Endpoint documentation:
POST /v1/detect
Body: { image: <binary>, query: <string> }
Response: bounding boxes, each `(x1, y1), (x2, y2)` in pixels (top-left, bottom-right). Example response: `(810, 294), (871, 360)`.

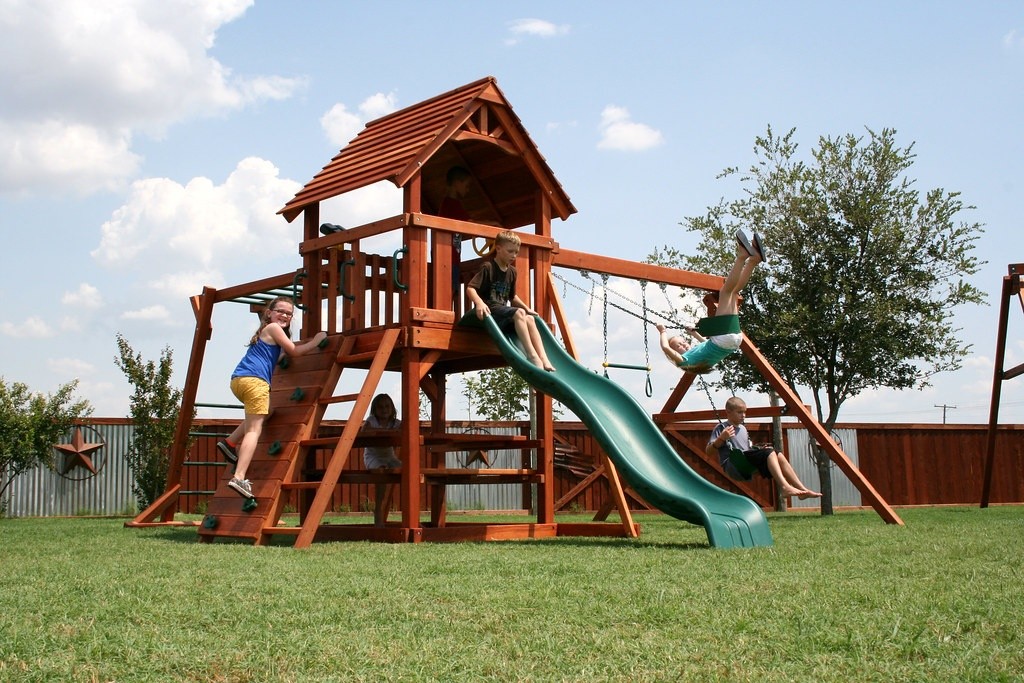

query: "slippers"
(752, 233), (766, 262)
(736, 229), (755, 256)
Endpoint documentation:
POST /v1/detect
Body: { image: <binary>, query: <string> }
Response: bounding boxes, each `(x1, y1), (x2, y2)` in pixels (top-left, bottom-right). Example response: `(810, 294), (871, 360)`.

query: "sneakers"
(227, 478), (255, 499)
(217, 439), (238, 464)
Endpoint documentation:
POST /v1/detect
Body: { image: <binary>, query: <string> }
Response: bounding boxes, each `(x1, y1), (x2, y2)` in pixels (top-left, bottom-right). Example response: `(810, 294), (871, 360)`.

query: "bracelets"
(718, 437), (725, 443)
(661, 329), (667, 333)
(712, 443), (719, 450)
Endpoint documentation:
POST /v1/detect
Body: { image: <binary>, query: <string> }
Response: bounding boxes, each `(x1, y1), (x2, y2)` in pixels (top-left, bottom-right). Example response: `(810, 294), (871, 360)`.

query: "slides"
(459, 303), (774, 549)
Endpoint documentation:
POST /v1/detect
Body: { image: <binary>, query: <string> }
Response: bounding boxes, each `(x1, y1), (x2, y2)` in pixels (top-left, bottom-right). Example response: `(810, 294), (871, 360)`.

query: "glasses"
(271, 310), (293, 318)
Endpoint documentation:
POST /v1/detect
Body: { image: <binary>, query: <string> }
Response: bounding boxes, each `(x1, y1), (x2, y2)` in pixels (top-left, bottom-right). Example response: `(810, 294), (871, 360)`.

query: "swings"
(550, 269), (742, 337)
(658, 284), (758, 482)
(602, 273), (652, 371)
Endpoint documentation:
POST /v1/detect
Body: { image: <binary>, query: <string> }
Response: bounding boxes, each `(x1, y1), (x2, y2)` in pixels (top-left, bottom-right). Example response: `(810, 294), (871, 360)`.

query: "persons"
(654, 228), (767, 373)
(362, 393), (408, 525)
(465, 230), (557, 372)
(216, 295), (327, 499)
(703, 397), (823, 500)
